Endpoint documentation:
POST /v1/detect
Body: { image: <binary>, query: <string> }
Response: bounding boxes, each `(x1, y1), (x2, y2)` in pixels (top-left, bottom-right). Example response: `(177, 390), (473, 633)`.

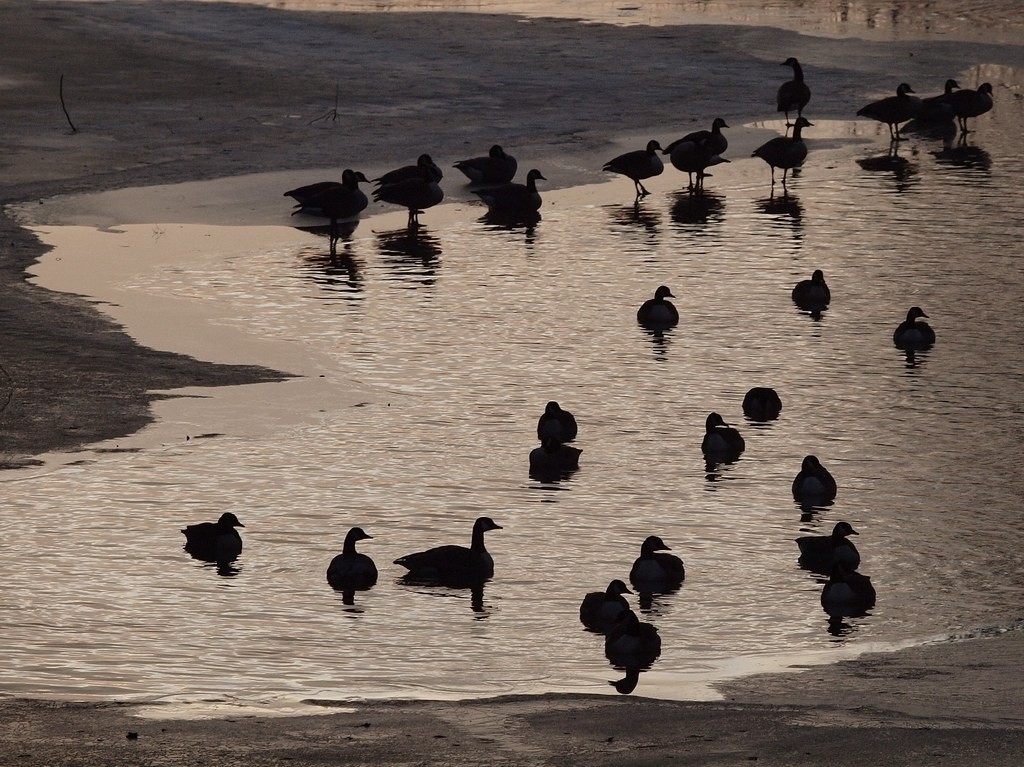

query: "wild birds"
(601, 140), (665, 200)
(371, 154), (445, 214)
(629, 533), (687, 597)
(742, 387), (783, 420)
(792, 269), (831, 311)
(637, 285), (680, 328)
(792, 521), (862, 574)
(792, 455), (838, 506)
(283, 168), (372, 220)
(577, 580), (639, 632)
(663, 117), (731, 191)
(603, 624), (663, 697)
(452, 144), (547, 217)
(893, 306), (936, 350)
(701, 413), (745, 463)
(819, 575), (878, 638)
(856, 78), (995, 142)
(327, 528), (379, 590)
(178, 512), (247, 563)
(392, 515), (506, 581)
(776, 57), (816, 128)
(537, 401), (578, 443)
(750, 117), (815, 193)
